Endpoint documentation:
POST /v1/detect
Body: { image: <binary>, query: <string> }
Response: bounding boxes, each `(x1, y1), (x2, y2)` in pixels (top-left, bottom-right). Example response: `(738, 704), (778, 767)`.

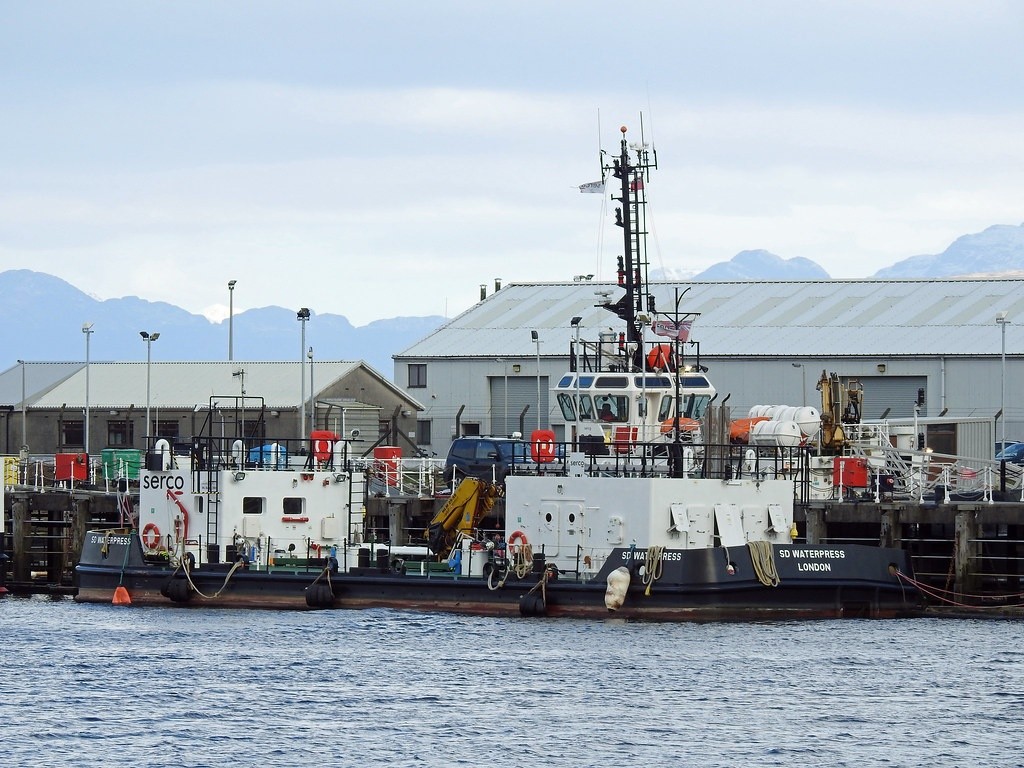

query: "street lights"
(570, 316), (583, 453)
(296, 306), (310, 451)
(138, 331), (160, 453)
(82, 320), (95, 454)
(991, 309), (1012, 462)
(233, 369), (246, 438)
(226, 279), (238, 360)
(531, 330), (544, 431)
(16, 359), (26, 447)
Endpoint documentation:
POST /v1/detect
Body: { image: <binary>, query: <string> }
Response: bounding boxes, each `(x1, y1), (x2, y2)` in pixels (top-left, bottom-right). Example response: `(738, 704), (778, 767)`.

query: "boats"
(74, 104), (1022, 622)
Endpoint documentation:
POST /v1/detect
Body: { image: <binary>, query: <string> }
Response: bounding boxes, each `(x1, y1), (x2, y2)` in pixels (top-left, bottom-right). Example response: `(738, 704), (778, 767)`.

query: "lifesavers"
(142, 522), (161, 550)
(303, 585), (333, 609)
(389, 558), (406, 578)
(507, 530), (528, 556)
